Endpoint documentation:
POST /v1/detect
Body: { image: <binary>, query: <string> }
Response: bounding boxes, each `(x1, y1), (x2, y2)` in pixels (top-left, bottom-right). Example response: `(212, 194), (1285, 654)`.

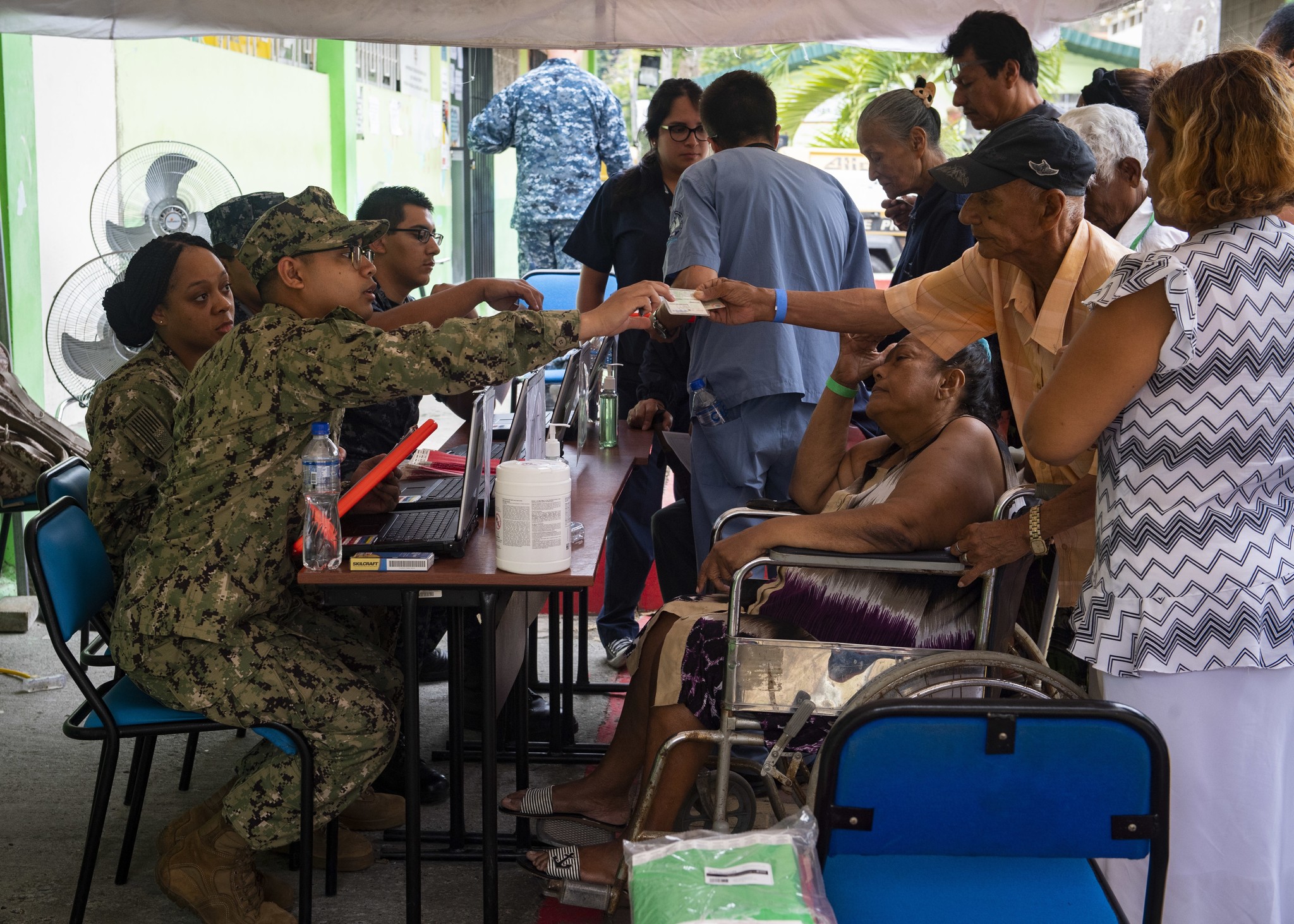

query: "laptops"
(342, 335), (614, 556)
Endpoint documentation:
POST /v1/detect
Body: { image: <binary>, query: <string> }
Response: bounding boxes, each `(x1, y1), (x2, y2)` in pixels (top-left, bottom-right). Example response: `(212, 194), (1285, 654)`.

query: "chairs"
(24, 456), (338, 924)
(511, 269), (617, 413)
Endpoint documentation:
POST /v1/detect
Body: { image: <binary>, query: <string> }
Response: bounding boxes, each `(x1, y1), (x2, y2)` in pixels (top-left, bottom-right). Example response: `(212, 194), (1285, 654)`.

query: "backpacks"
(0, 340), (92, 509)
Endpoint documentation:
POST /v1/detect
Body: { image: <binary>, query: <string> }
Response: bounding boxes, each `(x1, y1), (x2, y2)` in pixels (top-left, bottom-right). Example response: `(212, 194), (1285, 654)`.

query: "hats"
(204, 191), (292, 249)
(235, 185), (389, 287)
(928, 114), (1098, 197)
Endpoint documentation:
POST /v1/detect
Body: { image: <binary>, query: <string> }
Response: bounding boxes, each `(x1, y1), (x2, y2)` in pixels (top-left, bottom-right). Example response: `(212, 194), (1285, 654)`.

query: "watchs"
(1029, 504), (1050, 557)
(653, 308), (677, 339)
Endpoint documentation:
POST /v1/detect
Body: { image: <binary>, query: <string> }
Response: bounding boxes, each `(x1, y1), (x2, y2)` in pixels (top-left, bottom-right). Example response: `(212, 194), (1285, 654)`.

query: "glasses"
(944, 55), (1010, 83)
(706, 134), (718, 143)
(660, 124), (709, 141)
(388, 227), (444, 246)
(290, 243), (375, 271)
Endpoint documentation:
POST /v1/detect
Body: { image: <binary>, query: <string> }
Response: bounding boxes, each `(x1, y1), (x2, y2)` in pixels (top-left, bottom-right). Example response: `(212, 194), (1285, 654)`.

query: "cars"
(508, 267), (625, 419)
(857, 231), (903, 295)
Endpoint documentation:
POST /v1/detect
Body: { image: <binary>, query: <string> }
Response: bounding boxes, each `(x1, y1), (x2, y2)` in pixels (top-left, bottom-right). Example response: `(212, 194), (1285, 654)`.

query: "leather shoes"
(372, 731), (450, 804)
(419, 647), (448, 681)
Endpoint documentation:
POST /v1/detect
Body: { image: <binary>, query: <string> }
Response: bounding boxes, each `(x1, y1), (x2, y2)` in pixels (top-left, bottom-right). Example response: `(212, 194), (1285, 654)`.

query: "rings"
(956, 540), (965, 554)
(962, 553), (969, 563)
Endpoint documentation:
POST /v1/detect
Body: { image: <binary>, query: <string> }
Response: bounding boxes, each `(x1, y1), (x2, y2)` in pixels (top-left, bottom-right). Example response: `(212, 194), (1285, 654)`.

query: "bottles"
(603, 347), (612, 368)
(689, 378), (727, 427)
(590, 336), (604, 370)
(301, 423), (342, 570)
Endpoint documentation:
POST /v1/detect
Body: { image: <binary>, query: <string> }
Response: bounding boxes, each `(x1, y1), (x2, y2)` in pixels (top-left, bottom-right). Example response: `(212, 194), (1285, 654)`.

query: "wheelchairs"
(535, 479), (1108, 915)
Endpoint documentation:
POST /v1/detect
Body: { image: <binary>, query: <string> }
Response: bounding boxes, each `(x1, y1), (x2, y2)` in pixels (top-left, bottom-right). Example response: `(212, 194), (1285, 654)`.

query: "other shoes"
(269, 821), (375, 871)
(338, 786), (406, 830)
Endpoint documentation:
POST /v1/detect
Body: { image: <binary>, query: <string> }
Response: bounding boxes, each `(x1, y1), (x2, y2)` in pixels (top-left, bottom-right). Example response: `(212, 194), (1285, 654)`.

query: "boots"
(153, 809), (299, 924)
(157, 774), (295, 912)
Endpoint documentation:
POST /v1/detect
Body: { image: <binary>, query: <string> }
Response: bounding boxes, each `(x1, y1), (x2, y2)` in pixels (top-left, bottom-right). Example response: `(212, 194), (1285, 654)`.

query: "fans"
(47, 141), (243, 408)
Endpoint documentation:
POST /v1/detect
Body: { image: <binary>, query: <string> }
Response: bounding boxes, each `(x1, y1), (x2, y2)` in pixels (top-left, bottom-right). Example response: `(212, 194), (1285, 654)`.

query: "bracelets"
(773, 288), (788, 324)
(825, 376), (860, 399)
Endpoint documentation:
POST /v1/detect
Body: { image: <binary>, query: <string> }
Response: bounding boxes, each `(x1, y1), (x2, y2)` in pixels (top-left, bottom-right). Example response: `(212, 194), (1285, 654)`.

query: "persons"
(463, 1), (1294, 923)
(86, 181), (676, 924)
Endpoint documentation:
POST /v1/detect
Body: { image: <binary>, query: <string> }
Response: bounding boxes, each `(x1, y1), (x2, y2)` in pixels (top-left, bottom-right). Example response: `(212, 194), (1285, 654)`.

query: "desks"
(297, 420), (654, 924)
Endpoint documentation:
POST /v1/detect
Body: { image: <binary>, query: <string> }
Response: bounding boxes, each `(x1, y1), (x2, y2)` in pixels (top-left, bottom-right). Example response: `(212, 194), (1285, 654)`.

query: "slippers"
(498, 785), (628, 833)
(517, 845), (629, 894)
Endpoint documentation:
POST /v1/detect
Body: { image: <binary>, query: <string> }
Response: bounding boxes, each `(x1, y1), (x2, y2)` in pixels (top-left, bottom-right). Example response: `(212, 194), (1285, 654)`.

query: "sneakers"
(604, 636), (634, 668)
